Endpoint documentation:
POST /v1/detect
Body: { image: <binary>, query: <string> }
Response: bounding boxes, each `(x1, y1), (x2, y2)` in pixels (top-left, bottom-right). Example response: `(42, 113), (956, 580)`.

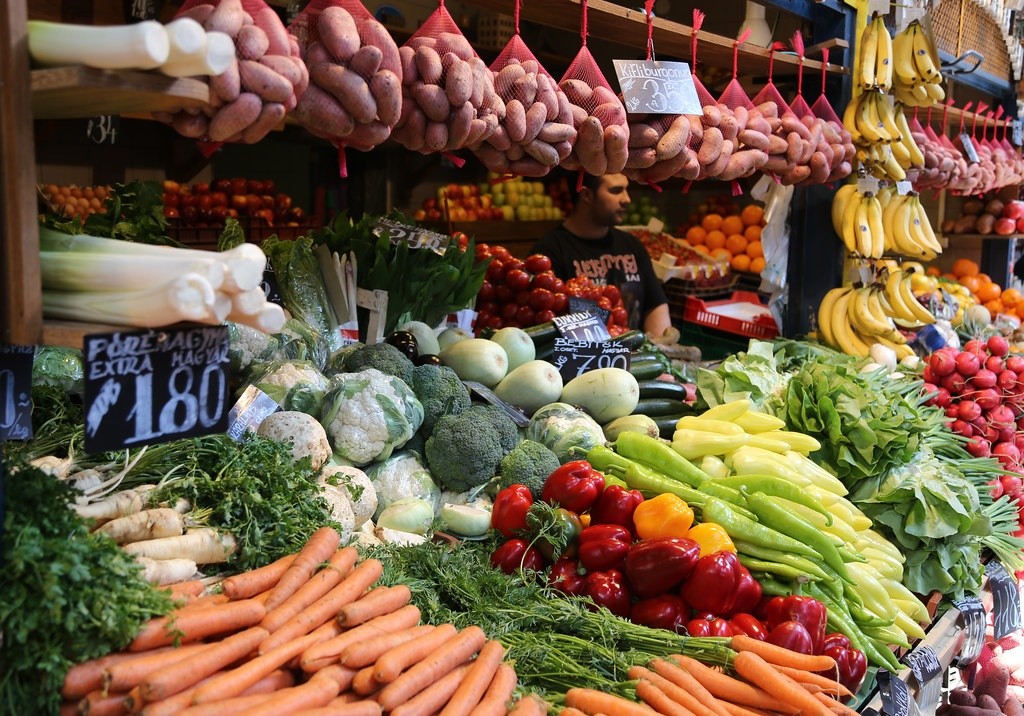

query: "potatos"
(942, 197), (1004, 235)
(936, 628), (1024, 716)
(909, 132), (1024, 196)
(168, 0), (855, 186)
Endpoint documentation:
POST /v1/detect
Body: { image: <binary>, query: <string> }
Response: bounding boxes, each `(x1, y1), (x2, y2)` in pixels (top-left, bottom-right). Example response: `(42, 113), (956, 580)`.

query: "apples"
(163, 179), (305, 225)
(413, 174), (573, 222)
(620, 195), (740, 238)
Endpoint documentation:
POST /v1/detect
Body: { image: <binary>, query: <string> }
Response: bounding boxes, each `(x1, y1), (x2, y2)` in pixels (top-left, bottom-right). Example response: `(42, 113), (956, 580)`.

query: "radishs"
(10, 456), (236, 589)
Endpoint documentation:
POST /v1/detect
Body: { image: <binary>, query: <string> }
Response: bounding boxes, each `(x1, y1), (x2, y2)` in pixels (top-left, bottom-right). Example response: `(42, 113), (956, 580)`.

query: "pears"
(41, 182), (112, 220)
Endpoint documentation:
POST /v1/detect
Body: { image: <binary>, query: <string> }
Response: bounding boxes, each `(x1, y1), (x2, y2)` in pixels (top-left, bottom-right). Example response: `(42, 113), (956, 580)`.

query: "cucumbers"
(515, 321), (714, 440)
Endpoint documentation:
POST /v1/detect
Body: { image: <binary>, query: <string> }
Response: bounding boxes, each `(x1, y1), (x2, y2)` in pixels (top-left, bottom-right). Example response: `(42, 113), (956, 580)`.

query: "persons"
(526, 170), (671, 337)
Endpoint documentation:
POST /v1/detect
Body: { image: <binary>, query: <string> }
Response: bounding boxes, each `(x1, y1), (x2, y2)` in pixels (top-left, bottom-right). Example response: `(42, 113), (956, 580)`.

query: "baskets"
(682, 291), (781, 340)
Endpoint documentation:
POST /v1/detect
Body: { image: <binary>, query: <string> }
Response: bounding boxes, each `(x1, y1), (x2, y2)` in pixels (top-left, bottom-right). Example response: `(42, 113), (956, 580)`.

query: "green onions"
(26, 19), (234, 77)
(38, 226), (286, 334)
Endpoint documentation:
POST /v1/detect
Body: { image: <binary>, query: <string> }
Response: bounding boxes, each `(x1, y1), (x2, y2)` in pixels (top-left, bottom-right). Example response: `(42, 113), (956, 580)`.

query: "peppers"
(493, 399), (934, 701)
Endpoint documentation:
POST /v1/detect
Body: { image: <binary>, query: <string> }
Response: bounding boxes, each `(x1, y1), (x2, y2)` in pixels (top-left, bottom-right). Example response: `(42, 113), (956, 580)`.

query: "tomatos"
(449, 231), (629, 339)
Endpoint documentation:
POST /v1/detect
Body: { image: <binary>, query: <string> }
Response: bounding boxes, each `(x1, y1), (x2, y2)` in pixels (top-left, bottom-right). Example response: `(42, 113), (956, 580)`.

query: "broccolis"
(227, 323), (561, 551)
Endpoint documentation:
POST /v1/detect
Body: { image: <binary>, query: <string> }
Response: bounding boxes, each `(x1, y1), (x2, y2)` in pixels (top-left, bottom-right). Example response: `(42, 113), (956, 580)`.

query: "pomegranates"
(993, 196), (1024, 236)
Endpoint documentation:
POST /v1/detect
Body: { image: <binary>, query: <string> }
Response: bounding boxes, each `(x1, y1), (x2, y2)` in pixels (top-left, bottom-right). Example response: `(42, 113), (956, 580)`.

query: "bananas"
(814, 9), (944, 361)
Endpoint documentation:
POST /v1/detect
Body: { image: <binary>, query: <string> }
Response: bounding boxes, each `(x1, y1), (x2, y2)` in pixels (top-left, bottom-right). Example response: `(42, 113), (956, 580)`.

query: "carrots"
(59, 527), (859, 716)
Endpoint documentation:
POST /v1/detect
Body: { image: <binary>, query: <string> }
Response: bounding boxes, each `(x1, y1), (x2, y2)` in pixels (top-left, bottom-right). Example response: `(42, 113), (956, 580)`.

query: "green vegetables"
(692, 338), (1024, 601)
(47, 177), (189, 249)
(217, 208), (493, 346)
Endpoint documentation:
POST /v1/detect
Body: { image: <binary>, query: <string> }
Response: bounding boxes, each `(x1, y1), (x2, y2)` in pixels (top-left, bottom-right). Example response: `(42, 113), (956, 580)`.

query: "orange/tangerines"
(933, 258), (1024, 341)
(676, 205), (767, 277)
